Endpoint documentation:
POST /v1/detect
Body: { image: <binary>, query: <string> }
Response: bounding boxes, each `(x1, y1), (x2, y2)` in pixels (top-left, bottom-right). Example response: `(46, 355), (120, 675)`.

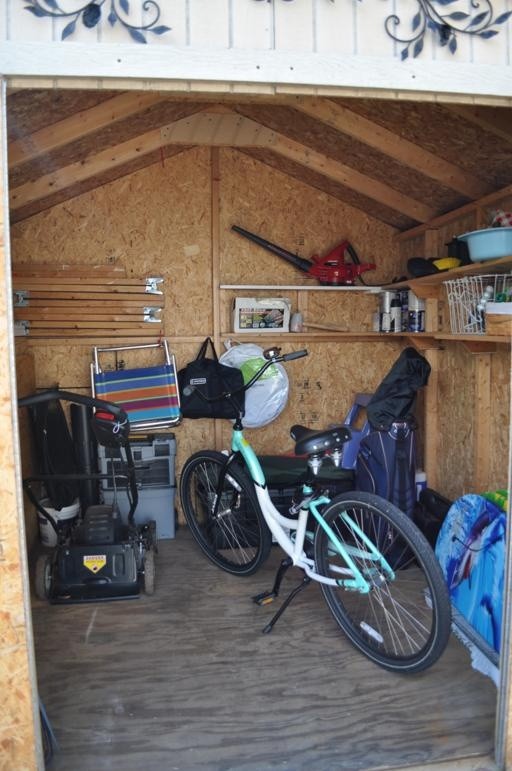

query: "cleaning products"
(17, 390), (155, 605)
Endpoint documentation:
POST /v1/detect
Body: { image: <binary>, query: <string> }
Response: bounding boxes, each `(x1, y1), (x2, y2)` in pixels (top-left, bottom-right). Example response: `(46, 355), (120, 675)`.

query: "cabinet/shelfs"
(390, 299), (402, 332)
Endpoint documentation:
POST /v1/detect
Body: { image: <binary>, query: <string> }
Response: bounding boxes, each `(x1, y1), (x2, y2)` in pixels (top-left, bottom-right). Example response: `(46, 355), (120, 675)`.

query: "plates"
(218, 255), (512, 349)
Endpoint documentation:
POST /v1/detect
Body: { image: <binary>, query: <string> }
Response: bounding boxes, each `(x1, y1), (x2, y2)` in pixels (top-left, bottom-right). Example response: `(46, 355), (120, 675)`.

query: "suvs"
(35, 499), (80, 547)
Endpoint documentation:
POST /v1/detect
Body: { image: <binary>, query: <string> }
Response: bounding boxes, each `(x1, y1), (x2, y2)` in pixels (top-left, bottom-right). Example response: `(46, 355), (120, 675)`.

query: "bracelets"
(456, 227), (512, 264)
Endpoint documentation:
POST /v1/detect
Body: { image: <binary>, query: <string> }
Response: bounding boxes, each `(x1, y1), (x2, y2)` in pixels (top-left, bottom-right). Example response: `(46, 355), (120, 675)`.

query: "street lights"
(177, 336), (247, 421)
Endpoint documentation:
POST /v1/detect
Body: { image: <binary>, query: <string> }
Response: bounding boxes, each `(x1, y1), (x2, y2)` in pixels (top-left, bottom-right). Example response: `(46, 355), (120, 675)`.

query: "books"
(204, 484), (340, 551)
(96, 432), (178, 542)
